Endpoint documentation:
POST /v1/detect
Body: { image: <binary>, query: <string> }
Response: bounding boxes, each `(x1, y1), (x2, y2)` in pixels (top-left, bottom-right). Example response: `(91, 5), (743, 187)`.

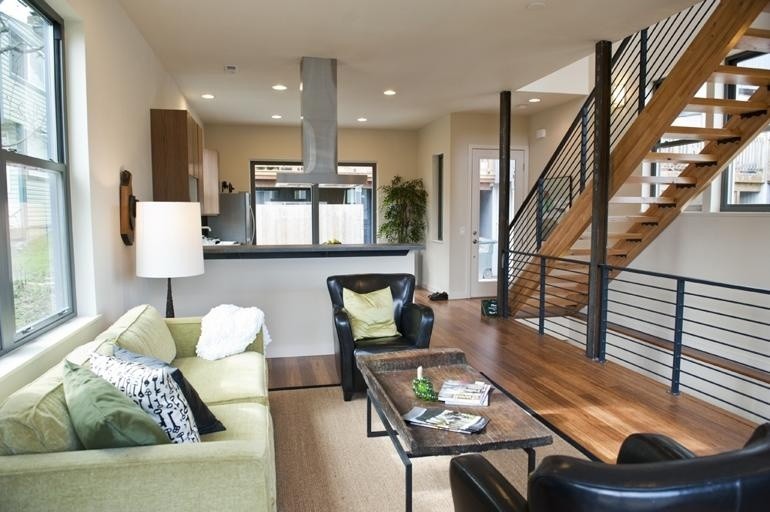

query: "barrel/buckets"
(481, 299), (497, 316)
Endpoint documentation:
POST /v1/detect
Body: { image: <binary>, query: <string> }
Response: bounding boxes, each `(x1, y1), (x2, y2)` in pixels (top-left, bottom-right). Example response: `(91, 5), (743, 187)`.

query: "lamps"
(131, 199), (209, 316)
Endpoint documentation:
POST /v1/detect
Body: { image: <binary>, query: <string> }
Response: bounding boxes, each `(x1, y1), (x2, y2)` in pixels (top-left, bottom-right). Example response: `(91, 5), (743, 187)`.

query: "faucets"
(202, 225), (212, 232)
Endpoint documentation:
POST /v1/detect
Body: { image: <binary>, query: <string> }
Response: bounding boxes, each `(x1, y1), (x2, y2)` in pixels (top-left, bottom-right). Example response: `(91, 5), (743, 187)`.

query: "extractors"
(276, 56), (367, 190)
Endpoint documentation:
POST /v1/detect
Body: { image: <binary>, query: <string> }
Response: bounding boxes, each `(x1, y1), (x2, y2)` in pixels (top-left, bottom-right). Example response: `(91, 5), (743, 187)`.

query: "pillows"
(339, 278), (404, 343)
(87, 345), (202, 442)
(58, 354), (168, 450)
(109, 339), (227, 434)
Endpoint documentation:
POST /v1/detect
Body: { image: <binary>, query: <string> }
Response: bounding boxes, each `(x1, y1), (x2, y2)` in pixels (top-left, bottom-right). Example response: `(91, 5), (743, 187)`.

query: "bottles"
(414, 366), (438, 401)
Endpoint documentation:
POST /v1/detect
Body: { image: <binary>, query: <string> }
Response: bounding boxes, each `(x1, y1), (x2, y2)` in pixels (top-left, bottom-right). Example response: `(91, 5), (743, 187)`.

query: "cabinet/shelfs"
(150, 109), (205, 217)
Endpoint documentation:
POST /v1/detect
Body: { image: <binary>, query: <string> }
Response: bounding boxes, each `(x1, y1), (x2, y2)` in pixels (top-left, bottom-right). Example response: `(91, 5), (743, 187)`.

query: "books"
(401, 407), (490, 435)
(437, 379), (491, 406)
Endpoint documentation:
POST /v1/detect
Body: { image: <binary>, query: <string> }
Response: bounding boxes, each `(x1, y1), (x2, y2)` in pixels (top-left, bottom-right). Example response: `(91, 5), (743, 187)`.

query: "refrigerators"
(210, 191), (254, 246)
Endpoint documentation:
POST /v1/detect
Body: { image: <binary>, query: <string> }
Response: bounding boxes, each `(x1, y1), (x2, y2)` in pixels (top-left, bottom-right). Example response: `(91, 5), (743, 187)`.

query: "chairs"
(448, 416), (770, 510)
(325, 271), (436, 401)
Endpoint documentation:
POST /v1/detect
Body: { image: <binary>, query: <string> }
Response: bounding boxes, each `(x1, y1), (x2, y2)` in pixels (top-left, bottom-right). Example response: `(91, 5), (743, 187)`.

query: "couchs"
(1, 300), (278, 512)
